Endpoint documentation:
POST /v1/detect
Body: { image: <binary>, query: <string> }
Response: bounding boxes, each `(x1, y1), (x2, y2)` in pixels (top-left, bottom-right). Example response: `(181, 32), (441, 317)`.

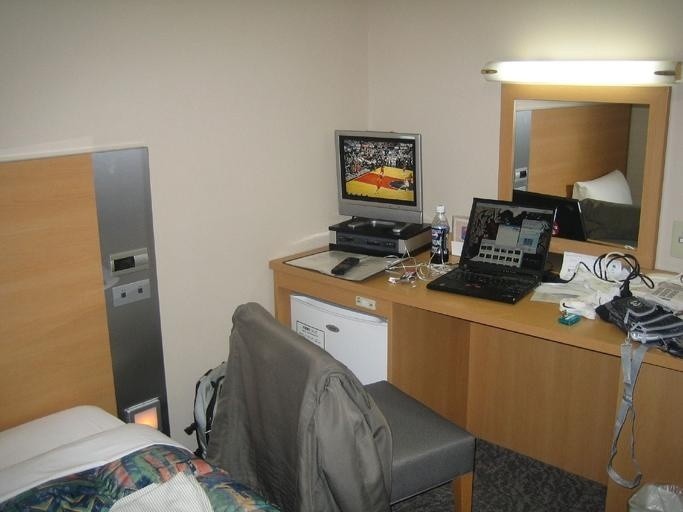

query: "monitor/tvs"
(334, 129), (423, 233)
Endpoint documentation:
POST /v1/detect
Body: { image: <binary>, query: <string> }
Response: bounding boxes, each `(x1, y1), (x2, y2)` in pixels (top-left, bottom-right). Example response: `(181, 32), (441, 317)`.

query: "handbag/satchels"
(595, 297), (683, 357)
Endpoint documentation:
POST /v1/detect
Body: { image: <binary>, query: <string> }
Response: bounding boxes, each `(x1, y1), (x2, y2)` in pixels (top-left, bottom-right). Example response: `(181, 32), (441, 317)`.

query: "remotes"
(331, 257), (360, 276)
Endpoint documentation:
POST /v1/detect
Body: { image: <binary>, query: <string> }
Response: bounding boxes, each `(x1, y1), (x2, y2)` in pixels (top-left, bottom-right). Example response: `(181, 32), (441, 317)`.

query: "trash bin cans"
(627, 482), (683, 512)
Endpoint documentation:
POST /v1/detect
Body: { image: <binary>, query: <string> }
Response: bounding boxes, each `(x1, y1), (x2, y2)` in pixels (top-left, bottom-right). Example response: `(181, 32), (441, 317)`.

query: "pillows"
(569, 168), (634, 205)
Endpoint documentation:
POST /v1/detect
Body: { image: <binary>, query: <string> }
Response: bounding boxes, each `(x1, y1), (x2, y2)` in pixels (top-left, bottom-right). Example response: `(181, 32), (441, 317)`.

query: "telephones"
(641, 273), (683, 313)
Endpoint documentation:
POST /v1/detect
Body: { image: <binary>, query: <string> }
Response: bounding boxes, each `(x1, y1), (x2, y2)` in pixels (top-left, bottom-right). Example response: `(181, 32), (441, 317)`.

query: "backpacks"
(184, 361), (227, 460)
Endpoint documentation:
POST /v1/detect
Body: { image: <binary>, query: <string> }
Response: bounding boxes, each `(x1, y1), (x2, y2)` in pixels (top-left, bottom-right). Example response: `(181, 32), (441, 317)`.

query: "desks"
(267, 242), (683, 507)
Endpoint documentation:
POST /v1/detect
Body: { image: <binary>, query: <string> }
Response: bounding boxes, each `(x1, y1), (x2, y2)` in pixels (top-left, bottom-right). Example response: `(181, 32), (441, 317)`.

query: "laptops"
(426, 197), (558, 304)
(512, 189), (587, 241)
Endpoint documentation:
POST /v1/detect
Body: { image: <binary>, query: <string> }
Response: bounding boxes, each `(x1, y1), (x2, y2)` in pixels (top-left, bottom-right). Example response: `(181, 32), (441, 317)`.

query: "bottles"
(431, 205), (449, 264)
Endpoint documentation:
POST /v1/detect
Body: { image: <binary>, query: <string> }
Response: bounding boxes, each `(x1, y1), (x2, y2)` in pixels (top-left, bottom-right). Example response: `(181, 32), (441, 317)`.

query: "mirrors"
(497, 82), (672, 269)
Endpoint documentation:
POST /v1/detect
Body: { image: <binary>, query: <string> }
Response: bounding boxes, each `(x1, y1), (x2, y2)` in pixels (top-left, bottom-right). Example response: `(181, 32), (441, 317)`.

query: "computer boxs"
(328, 217), (433, 258)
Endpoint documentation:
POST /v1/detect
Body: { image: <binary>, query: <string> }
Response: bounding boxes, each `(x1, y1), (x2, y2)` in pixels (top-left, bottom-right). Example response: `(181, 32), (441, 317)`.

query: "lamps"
(481, 59), (683, 86)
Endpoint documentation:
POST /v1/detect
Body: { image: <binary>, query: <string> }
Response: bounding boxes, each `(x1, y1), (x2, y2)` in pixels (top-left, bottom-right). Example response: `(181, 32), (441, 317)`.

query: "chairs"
(577, 199), (640, 245)
(234, 300), (476, 512)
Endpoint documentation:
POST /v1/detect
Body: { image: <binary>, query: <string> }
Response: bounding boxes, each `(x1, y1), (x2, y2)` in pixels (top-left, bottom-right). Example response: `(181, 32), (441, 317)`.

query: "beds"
(1, 403), (280, 512)
